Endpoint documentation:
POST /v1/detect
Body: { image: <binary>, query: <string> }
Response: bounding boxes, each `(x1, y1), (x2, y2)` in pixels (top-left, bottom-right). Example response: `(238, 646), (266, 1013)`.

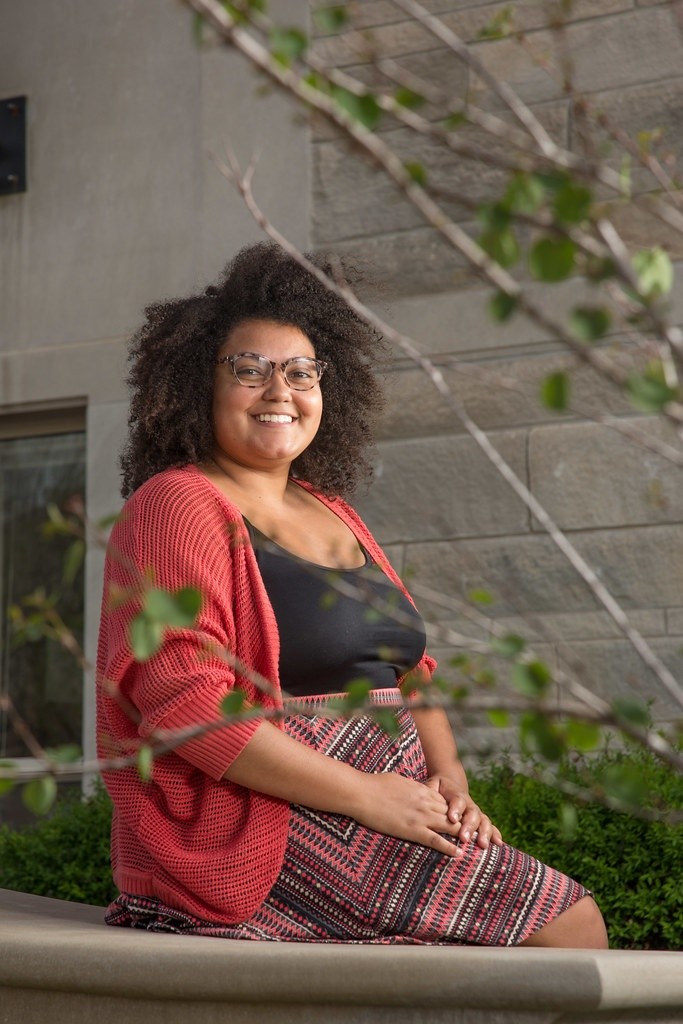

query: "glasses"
(212, 352), (328, 391)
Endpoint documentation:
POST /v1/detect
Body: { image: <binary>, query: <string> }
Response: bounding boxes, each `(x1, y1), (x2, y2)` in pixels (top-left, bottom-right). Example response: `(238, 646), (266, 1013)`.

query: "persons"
(96, 242), (610, 949)
